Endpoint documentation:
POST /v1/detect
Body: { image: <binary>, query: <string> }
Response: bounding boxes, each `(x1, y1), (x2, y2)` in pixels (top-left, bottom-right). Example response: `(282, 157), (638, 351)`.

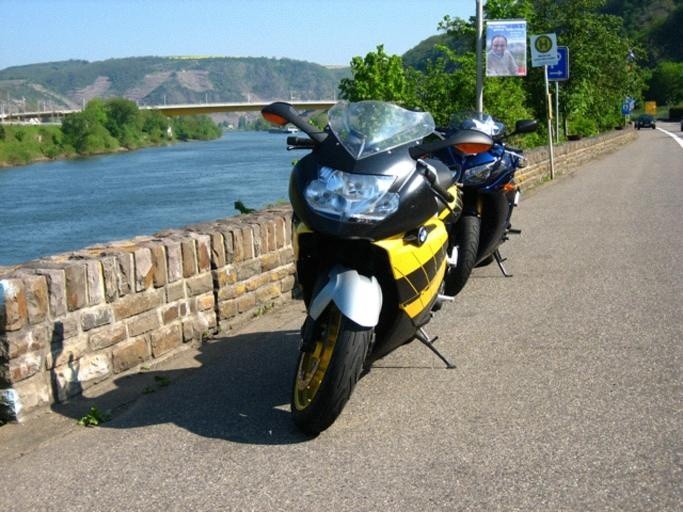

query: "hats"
(635, 115), (656, 130)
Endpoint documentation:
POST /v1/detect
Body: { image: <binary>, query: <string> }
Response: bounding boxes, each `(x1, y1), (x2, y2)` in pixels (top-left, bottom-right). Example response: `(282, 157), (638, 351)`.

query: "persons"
(487, 34), (520, 76)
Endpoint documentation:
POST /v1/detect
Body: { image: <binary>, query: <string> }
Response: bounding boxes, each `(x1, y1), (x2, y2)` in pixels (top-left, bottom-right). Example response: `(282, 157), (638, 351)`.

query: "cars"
(635, 115), (656, 130)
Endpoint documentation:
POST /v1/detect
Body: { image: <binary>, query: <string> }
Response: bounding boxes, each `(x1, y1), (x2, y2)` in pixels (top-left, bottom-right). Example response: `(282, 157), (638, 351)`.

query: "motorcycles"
(261, 100), (463, 436)
(422, 111), (539, 298)
